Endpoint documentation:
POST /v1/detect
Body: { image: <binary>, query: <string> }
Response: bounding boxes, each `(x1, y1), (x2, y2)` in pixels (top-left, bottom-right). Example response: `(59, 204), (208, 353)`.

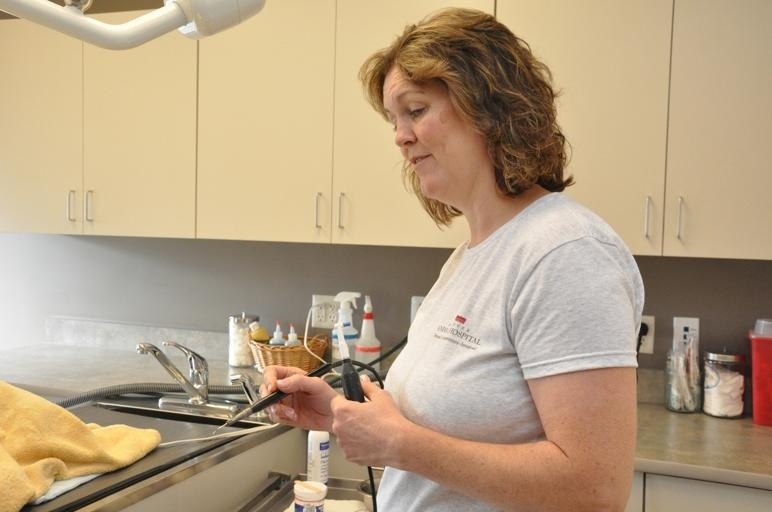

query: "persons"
(251, 7), (643, 510)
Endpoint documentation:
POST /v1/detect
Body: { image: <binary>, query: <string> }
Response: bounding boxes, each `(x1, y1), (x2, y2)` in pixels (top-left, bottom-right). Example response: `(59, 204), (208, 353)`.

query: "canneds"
(703, 350), (745, 418)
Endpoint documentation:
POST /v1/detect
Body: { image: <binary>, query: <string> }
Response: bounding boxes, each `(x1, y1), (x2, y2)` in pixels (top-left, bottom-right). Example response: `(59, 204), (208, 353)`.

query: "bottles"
(293, 427), (332, 511)
(703, 350), (746, 420)
(228, 310), (261, 369)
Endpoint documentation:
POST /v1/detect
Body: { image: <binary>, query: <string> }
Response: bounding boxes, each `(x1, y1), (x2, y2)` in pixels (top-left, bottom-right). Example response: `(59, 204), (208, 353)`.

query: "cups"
(663, 350), (702, 415)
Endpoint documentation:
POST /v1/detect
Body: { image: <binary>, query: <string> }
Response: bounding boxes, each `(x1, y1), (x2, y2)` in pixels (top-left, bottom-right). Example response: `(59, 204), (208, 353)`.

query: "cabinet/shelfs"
(195, 0), (495, 251)
(0, 4), (196, 244)
(496, 0), (772, 259)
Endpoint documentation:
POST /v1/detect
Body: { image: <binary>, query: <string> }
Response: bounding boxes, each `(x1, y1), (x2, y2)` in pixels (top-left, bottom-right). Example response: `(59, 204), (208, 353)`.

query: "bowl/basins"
(356, 477), (381, 511)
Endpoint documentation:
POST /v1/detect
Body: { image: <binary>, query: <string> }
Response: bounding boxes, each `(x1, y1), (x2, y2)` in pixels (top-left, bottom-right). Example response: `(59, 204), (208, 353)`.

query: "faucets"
(136, 338), (210, 405)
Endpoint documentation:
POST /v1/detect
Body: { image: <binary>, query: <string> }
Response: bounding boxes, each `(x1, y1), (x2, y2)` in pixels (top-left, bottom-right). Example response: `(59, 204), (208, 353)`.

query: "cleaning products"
(328, 289), (361, 374)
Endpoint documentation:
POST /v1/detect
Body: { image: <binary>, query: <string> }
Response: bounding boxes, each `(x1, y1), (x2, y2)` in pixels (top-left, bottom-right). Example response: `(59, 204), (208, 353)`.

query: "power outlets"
(310, 294), (341, 330)
(637, 316), (655, 355)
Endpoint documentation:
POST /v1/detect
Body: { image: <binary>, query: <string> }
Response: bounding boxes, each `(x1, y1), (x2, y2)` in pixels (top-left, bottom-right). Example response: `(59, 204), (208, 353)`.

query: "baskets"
(247, 329), (331, 375)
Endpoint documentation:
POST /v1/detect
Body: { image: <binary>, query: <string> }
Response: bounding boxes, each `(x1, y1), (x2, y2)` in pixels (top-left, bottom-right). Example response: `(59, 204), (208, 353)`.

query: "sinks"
(90, 400), (264, 431)
